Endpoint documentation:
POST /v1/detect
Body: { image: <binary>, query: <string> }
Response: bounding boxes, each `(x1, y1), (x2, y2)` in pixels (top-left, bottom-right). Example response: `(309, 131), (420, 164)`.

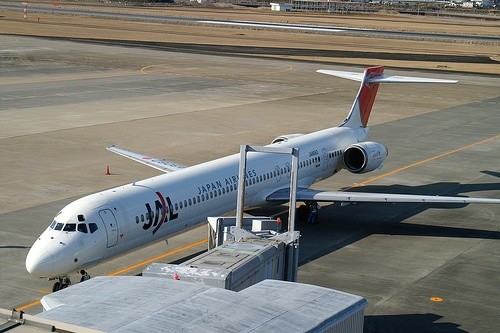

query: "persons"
(80, 270), (90, 282)
(307, 201), (319, 223)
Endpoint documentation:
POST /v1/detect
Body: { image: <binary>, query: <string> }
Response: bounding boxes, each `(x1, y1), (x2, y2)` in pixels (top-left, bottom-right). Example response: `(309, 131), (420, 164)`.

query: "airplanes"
(25, 65), (500, 292)
